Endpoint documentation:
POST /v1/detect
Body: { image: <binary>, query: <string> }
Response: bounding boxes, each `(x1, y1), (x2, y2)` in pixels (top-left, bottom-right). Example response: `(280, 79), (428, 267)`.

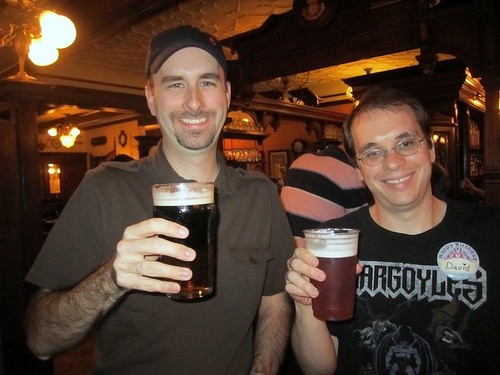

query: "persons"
(284, 87), (500, 375)
(23, 25), (297, 375)
(279, 136), (370, 238)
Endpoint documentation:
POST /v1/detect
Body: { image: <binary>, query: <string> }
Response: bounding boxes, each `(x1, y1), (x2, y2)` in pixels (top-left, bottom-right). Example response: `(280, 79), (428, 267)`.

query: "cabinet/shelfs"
(217, 127), (271, 179)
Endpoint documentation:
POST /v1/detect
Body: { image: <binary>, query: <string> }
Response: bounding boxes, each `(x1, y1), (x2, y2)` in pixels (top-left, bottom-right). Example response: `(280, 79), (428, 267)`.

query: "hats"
(145, 25), (228, 77)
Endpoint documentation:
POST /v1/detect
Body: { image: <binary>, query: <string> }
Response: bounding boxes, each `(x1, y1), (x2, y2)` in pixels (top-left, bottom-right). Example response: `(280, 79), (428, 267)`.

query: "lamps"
(0, 0), (77, 83)
(47, 114), (80, 149)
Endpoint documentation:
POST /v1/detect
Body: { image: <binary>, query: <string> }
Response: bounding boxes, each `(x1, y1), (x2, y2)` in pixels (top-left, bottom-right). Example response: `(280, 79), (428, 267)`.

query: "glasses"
(355, 136), (427, 166)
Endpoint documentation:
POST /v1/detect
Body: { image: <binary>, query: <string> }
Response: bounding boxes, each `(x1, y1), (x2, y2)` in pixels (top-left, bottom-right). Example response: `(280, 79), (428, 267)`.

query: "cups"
(304, 228), (359, 321)
(151, 181), (215, 299)
